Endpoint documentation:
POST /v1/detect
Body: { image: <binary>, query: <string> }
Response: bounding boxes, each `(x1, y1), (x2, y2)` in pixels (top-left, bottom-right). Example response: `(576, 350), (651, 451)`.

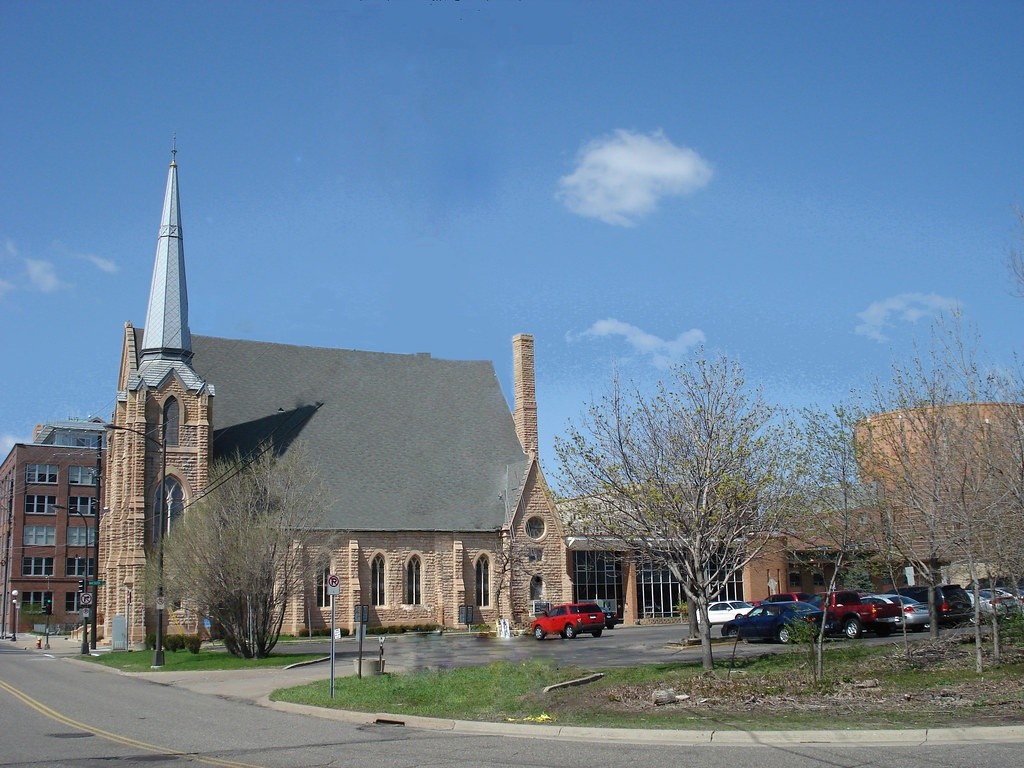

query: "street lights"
(51, 504), (91, 655)
(10, 589), (19, 642)
(102, 423), (166, 671)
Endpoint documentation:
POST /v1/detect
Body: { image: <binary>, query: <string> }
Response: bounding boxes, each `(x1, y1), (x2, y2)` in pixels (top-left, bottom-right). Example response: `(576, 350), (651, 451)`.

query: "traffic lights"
(78, 580), (85, 594)
(42, 606), (46, 615)
(46, 592), (55, 616)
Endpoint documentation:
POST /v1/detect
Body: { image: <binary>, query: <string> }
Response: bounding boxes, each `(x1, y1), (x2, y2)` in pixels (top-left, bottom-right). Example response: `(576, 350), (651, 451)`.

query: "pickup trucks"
(810, 590), (903, 640)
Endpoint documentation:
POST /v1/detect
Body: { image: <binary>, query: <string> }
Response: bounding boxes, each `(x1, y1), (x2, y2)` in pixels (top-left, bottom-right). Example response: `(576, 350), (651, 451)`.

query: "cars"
(752, 592), (811, 607)
(723, 602), (839, 646)
(977, 586), (1024, 617)
(695, 601), (775, 625)
(967, 593), (1008, 626)
(859, 594), (932, 633)
(600, 607), (618, 629)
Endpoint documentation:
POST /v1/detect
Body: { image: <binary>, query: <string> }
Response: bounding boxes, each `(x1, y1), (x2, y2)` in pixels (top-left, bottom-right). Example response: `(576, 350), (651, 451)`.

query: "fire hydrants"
(36, 637), (43, 649)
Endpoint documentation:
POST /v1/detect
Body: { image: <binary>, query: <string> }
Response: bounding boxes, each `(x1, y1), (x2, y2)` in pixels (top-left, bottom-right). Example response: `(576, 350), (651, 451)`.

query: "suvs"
(531, 602), (605, 639)
(883, 584), (974, 628)
